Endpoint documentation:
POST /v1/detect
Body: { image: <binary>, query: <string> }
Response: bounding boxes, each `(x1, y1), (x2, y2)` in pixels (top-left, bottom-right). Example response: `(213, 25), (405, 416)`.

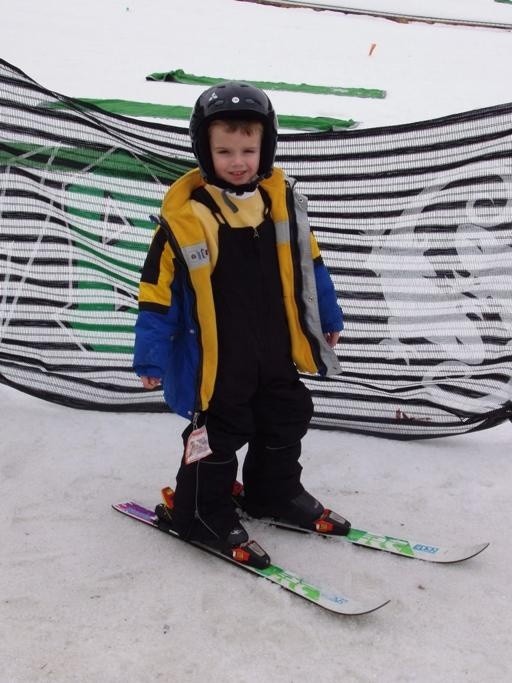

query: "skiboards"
(112, 478), (489, 616)
(34, 69), (386, 133)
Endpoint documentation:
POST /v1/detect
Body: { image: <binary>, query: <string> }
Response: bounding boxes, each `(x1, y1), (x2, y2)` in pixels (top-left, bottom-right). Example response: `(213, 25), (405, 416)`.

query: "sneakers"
(217, 489), (325, 543)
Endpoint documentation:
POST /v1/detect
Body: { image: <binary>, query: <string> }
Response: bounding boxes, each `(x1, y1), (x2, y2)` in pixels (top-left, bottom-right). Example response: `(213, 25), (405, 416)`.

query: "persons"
(131, 78), (344, 549)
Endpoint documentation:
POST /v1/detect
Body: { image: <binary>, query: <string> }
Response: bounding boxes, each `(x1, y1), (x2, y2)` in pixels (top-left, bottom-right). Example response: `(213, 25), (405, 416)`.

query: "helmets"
(189, 79), (279, 197)
